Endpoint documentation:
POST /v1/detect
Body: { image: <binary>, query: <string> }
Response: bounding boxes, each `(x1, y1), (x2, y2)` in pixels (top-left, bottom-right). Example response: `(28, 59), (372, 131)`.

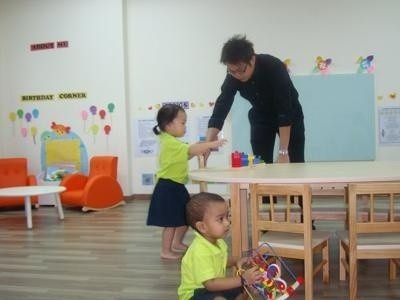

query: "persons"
(146, 103), (227, 261)
(196, 37), (316, 234)
(177, 191), (262, 300)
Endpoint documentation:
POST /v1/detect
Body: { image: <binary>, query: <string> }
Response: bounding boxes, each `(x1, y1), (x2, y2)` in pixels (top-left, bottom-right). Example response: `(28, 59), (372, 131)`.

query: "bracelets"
(279, 149), (288, 155)
(239, 275), (247, 292)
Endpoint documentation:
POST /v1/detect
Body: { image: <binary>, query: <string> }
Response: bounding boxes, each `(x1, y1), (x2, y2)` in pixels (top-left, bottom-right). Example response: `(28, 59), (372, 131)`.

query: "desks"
(0, 186), (67, 229)
(191, 162), (400, 257)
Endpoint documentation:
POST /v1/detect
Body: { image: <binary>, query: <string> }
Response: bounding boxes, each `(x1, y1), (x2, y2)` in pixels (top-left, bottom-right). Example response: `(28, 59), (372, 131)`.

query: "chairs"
(0, 157), (39, 209)
(199, 154), (231, 224)
(56, 156), (127, 212)
(250, 183), (331, 300)
(336, 183), (399, 298)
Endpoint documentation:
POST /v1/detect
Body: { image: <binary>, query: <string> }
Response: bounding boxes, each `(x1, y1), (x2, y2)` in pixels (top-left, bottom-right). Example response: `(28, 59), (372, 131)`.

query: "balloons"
(8, 108), (40, 137)
(80, 103), (115, 136)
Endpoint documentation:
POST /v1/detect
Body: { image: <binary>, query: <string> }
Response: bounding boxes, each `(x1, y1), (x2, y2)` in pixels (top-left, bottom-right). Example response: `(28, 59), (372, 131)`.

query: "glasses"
(226, 65), (248, 75)
